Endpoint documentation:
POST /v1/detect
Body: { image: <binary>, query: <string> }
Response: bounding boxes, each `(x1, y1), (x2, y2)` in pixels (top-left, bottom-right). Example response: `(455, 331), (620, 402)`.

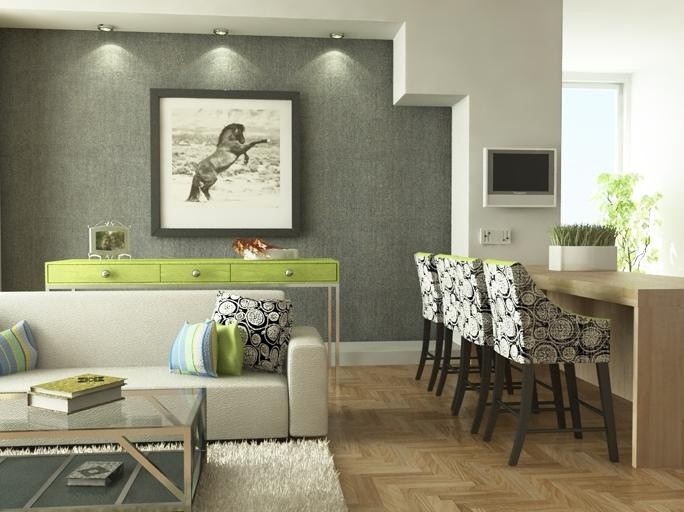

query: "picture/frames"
(88, 219), (132, 257)
(150, 88), (301, 237)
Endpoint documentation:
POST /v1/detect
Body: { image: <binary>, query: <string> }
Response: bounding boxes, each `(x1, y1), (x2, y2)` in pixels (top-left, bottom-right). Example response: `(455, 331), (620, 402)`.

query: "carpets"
(0, 438), (348, 512)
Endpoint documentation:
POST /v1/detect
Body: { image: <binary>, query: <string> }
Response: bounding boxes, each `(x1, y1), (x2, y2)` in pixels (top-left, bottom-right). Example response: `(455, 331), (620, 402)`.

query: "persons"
(108, 233), (121, 249)
(99, 235), (110, 249)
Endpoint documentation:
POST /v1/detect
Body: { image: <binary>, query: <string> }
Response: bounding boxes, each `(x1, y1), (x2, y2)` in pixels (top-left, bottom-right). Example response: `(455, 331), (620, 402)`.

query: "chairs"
(482, 258), (619, 466)
(451, 257), (566, 433)
(434, 253), (513, 411)
(415, 251), (483, 392)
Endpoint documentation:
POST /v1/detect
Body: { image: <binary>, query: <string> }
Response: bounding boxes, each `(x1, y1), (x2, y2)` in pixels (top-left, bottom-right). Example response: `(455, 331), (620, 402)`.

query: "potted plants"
(547, 224), (618, 272)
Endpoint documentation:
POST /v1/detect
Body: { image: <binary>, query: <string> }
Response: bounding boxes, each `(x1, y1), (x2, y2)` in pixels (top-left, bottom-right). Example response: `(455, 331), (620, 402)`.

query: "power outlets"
(480, 228), (512, 243)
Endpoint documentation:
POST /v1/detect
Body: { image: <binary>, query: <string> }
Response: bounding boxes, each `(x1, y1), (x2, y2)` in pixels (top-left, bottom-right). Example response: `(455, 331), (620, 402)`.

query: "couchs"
(0, 290), (328, 448)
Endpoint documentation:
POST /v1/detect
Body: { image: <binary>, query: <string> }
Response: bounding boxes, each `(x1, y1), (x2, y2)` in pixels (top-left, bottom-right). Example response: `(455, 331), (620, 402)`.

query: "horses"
(185, 122), (271, 201)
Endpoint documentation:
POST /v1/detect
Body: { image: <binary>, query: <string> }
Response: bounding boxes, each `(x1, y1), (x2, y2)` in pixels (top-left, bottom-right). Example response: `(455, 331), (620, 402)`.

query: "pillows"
(0, 321), (39, 376)
(169, 319), (218, 378)
(211, 290), (294, 376)
(206, 319), (243, 376)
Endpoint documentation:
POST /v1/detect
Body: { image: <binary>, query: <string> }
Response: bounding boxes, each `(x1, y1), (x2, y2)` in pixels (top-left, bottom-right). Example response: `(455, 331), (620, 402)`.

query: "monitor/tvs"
(484, 147), (558, 208)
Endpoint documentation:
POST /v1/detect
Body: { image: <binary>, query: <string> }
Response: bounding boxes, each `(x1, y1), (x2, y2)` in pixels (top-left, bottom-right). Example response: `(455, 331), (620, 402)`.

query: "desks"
(45, 258), (340, 383)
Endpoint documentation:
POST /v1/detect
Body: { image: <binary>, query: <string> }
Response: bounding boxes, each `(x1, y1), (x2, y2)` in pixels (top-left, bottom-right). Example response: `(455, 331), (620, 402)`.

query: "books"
(66, 459), (123, 486)
(27, 374), (126, 413)
